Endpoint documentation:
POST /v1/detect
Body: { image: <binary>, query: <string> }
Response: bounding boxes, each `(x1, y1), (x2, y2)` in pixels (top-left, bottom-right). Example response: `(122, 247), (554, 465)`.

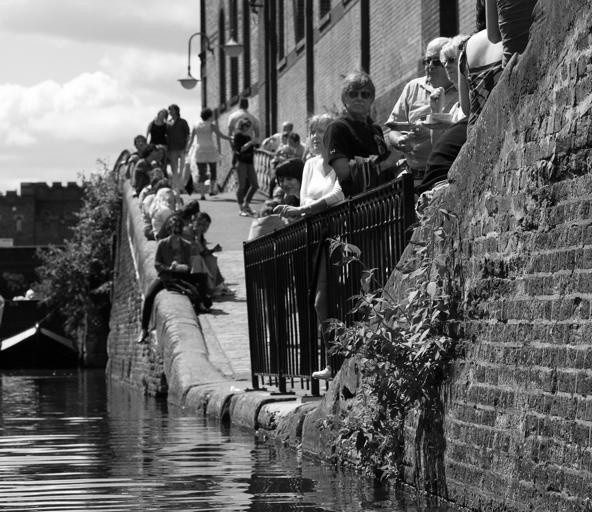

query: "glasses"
(349, 91), (370, 98)
(423, 59), (442, 66)
(444, 56), (455, 65)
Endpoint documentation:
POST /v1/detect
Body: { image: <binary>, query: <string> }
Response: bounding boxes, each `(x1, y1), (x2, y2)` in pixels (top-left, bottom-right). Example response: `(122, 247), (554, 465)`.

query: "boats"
(1, 300), (79, 369)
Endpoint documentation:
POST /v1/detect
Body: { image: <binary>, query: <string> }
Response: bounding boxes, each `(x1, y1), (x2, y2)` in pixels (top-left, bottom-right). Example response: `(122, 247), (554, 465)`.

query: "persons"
(226, 97), (263, 170)
(185, 108), (233, 199)
(456, 0), (504, 139)
(232, 116), (261, 218)
(418, 34), (471, 146)
(323, 68), (403, 294)
(256, 111), (347, 381)
(124, 135), (238, 344)
(484, 0), (537, 70)
(144, 107), (168, 146)
(413, 113), (468, 196)
(165, 104), (190, 176)
(381, 34), (459, 205)
